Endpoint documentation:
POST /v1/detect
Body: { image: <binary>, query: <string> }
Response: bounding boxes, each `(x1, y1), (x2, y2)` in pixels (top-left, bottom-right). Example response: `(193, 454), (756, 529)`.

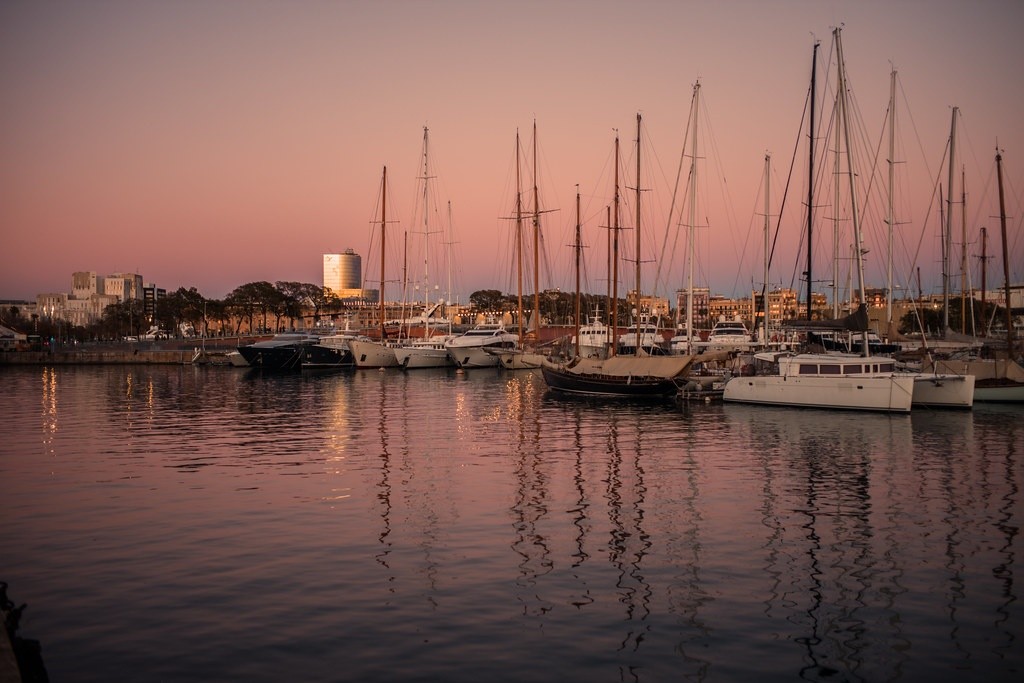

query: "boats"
(295, 333), (374, 364)
(234, 330), (326, 372)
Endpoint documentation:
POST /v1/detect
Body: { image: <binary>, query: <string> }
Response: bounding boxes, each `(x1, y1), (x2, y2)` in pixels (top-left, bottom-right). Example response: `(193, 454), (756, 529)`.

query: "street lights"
(203, 301), (209, 358)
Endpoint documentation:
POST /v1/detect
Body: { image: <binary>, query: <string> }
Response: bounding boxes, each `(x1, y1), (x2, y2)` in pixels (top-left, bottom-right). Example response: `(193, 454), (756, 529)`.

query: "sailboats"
(346, 21), (1024, 412)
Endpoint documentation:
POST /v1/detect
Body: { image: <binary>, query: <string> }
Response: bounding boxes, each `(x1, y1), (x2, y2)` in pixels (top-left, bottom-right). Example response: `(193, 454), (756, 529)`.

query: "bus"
(26, 335), (42, 350)
(0, 338), (28, 352)
